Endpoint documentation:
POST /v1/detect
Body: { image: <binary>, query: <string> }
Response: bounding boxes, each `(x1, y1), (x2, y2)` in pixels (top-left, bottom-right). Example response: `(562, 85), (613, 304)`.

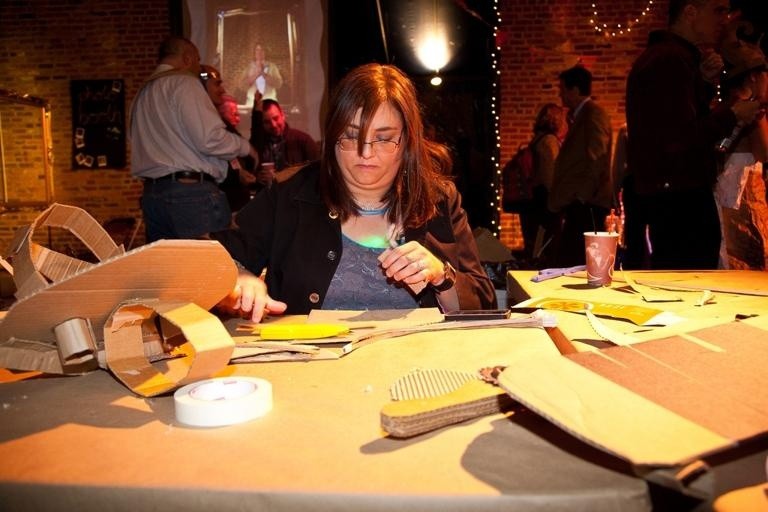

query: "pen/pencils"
(531, 264), (587, 282)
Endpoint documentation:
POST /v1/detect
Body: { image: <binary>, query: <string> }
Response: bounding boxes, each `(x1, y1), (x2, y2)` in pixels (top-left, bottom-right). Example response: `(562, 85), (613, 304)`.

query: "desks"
(499, 262), (768, 512)
(0, 300), (660, 511)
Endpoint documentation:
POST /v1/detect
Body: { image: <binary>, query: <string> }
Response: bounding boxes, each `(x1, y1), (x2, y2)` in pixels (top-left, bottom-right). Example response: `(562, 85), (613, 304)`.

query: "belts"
(142, 170), (218, 188)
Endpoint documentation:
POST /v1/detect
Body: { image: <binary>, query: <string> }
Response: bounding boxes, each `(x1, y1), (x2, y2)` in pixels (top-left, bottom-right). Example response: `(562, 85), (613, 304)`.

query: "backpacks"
(501, 132), (560, 214)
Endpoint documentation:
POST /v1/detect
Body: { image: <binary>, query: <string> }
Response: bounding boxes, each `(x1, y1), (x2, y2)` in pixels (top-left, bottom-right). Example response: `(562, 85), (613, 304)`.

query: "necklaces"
(356, 207), (388, 215)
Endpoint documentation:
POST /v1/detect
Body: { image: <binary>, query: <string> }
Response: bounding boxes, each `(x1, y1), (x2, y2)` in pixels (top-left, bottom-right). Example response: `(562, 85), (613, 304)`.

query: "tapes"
(171, 373), (274, 432)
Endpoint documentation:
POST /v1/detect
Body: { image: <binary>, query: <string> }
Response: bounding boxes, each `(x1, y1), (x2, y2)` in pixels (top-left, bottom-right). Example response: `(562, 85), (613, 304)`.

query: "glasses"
(334, 125), (403, 155)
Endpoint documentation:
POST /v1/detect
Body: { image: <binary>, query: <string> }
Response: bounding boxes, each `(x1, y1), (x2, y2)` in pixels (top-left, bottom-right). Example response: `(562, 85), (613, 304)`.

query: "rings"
(405, 253), (424, 271)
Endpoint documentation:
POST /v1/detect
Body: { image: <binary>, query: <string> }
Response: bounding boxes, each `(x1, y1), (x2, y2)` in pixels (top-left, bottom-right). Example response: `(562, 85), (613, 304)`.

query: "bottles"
(718, 121), (743, 153)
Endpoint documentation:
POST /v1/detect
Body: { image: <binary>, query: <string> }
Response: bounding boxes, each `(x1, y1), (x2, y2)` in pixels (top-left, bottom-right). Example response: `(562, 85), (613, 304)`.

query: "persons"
(548, 67), (614, 267)
(128, 35), (258, 244)
(217, 61), (498, 323)
(625, 2), (767, 270)
(615, 124), (628, 269)
(518, 104), (564, 259)
(242, 99), (320, 210)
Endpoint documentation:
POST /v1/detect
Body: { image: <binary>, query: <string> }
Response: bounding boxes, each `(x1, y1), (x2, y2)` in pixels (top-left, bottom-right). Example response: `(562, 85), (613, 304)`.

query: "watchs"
(445, 262), (458, 289)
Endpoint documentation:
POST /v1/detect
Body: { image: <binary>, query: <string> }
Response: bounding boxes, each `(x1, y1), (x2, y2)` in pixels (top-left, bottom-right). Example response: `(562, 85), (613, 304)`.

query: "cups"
(583, 231), (620, 285)
(262, 162), (275, 182)
